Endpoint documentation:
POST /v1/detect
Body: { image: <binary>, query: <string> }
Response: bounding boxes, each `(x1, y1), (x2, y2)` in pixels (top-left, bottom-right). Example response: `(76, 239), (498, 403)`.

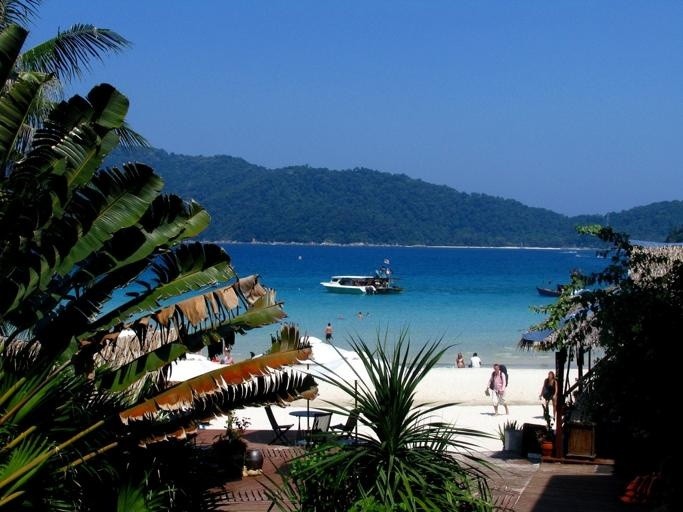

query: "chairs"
(485, 389), (490, 396)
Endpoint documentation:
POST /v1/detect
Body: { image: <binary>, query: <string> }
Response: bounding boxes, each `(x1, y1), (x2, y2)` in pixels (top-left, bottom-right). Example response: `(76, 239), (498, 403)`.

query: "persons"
(324, 323), (332, 344)
(487, 364), (509, 415)
(468, 352), (481, 368)
(358, 312), (362, 319)
(210, 348), (234, 364)
(456, 353), (464, 368)
(539, 371), (558, 417)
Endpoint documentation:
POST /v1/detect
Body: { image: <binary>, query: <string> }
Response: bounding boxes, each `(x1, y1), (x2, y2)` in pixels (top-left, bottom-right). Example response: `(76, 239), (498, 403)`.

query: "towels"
(504, 429), (524, 454)
(208, 340), (224, 362)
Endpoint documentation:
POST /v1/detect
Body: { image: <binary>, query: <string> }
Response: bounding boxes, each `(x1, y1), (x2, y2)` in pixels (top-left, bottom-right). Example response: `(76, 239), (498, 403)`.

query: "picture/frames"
(289, 410), (323, 444)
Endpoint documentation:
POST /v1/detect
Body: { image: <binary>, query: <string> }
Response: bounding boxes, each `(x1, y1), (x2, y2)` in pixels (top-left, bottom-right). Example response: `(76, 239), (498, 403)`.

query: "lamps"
(303, 407), (361, 452)
(264, 404), (294, 446)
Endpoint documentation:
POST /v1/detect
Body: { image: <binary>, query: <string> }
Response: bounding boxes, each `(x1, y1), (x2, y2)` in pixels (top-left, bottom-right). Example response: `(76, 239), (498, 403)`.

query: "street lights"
(543, 390), (553, 403)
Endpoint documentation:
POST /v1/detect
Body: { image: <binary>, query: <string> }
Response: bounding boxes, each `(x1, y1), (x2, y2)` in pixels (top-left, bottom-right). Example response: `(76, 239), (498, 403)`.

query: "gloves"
(318, 256), (404, 294)
(534, 284), (585, 298)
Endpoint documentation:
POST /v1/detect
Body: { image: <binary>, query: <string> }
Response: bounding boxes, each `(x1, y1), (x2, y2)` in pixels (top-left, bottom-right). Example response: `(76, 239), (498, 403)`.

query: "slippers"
(493, 365), (508, 387)
